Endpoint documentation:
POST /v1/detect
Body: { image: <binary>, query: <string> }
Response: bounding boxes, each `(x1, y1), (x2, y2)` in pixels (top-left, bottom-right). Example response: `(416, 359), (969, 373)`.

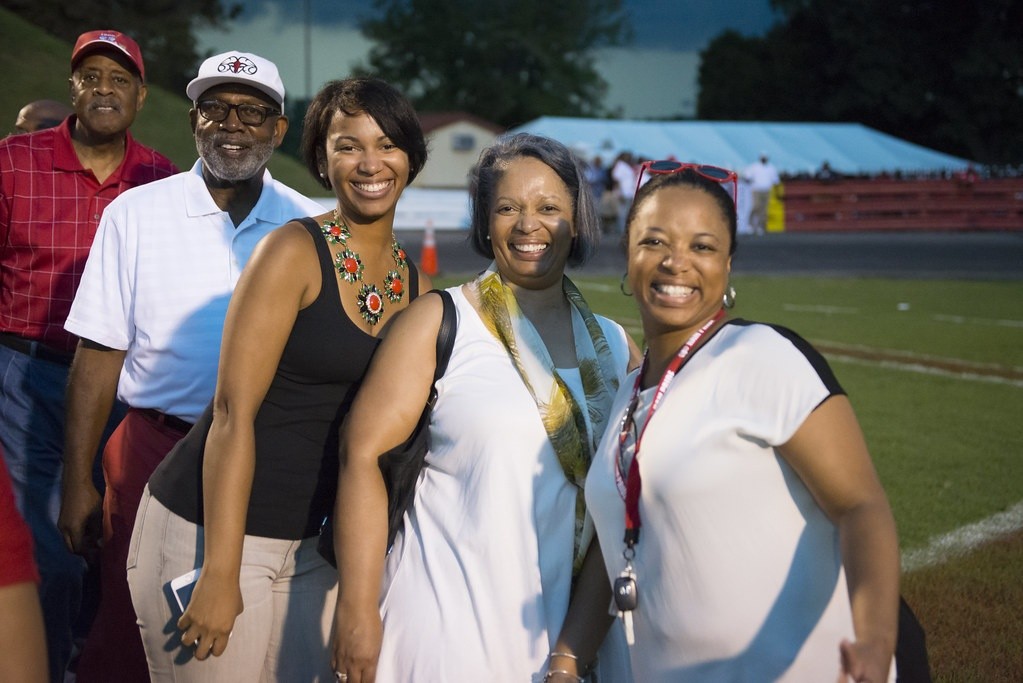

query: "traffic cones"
(420, 224), (439, 278)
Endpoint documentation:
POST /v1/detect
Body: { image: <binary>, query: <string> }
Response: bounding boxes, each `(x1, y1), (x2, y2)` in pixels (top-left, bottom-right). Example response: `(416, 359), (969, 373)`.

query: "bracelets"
(550, 652), (581, 662)
(543, 669), (585, 683)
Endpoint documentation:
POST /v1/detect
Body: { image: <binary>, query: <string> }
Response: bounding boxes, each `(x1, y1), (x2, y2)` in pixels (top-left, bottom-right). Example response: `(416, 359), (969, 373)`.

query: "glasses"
(634, 160), (738, 215)
(195, 100), (282, 126)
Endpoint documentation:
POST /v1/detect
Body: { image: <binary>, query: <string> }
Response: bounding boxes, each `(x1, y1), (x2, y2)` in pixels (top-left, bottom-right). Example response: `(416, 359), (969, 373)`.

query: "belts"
(0, 330), (77, 362)
(143, 407), (194, 435)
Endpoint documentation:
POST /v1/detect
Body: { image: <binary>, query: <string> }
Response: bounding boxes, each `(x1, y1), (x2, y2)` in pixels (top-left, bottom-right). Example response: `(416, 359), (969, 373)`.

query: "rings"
(334, 671), (347, 680)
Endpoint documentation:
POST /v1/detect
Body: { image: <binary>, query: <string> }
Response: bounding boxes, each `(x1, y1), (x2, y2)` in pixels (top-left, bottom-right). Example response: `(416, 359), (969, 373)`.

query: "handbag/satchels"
(317, 287), (457, 571)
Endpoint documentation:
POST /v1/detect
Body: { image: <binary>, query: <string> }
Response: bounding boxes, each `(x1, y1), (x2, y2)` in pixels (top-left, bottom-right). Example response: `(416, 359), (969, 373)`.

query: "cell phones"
(170, 567), (232, 649)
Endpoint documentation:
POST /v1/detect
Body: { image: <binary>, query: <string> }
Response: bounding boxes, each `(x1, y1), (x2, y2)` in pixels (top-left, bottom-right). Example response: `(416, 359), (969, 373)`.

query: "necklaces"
(321, 207), (407, 327)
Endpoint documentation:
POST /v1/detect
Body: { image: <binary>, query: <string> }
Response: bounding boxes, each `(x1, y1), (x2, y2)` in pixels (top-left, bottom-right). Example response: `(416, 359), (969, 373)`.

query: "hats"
(71, 30), (145, 85)
(186, 50), (284, 115)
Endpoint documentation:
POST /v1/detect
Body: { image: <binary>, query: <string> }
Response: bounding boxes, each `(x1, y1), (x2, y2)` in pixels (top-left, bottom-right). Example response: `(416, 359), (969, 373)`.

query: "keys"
(613, 576), (637, 646)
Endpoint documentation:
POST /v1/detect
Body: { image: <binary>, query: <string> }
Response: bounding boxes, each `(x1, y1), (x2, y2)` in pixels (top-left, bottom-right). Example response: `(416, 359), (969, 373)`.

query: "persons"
(742, 152), (782, 235)
(0, 30), (182, 683)
(0, 443), (51, 683)
(584, 149), (643, 234)
(126, 74), (432, 683)
(581, 167), (900, 683)
(14, 98), (72, 134)
(329, 138), (642, 682)
(55, 46), (330, 683)
